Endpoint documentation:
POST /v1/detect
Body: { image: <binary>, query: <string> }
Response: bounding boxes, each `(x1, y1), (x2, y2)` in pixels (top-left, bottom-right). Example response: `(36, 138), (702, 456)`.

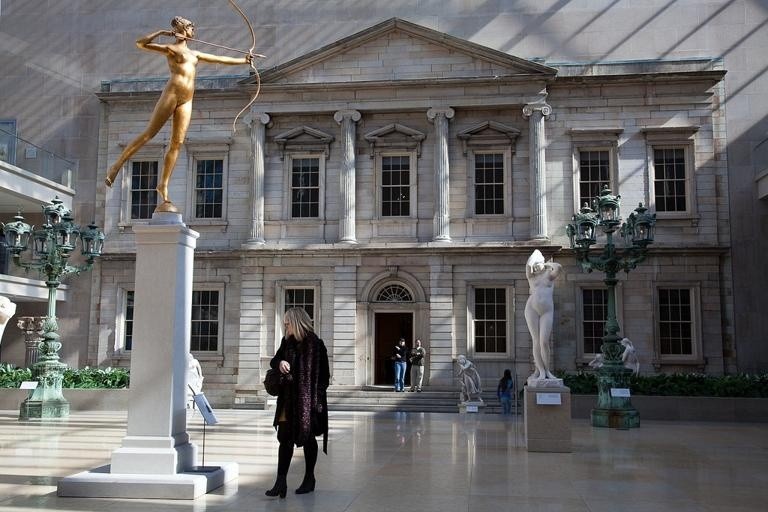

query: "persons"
(523, 248), (562, 380)
(390, 338), (409, 392)
(188, 351), (204, 378)
(105, 15), (253, 202)
(588, 345), (607, 369)
(620, 338), (641, 377)
(455, 355), (483, 402)
(263, 306), (330, 498)
(408, 339), (426, 393)
(496, 369), (513, 415)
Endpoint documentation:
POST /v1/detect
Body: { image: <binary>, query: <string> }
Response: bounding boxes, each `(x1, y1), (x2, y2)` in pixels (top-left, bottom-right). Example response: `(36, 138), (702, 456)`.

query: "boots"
(265, 476), (287, 498)
(296, 474), (315, 494)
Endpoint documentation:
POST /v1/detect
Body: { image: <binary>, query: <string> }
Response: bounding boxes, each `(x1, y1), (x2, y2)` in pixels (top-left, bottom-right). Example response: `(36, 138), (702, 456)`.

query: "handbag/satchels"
(263, 369), (293, 396)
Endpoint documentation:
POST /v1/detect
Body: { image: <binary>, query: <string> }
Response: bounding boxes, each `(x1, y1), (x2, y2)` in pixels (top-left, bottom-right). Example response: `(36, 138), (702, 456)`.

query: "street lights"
(565, 183), (659, 432)
(2, 195), (105, 420)
